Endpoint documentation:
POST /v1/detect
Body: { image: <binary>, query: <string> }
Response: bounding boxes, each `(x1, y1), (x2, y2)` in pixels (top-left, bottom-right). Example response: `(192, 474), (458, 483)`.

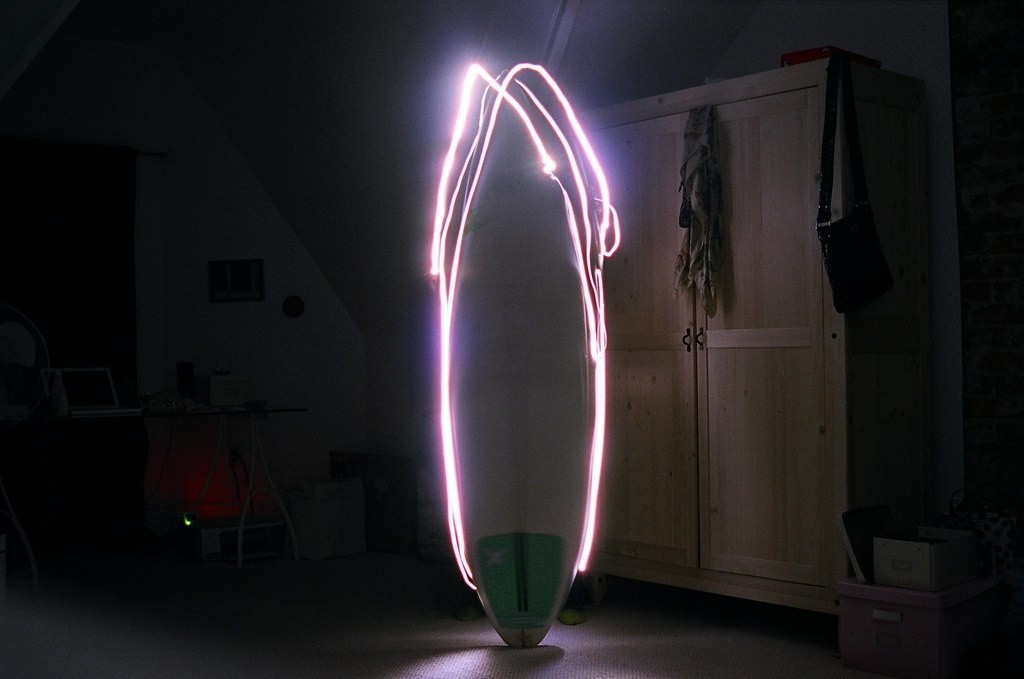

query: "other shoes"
(449, 597), (480, 621)
(560, 598), (587, 625)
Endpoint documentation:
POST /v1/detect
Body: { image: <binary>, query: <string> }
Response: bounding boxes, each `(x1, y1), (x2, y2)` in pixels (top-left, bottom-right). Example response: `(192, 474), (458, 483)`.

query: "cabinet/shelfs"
(589, 59), (924, 617)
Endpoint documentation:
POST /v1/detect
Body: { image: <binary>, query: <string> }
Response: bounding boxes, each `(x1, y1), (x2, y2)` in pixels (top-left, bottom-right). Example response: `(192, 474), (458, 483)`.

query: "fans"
(0, 303), (50, 429)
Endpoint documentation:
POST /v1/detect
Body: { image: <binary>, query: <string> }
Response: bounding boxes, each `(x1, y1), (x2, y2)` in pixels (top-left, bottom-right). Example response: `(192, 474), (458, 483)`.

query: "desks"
(0, 405), (309, 590)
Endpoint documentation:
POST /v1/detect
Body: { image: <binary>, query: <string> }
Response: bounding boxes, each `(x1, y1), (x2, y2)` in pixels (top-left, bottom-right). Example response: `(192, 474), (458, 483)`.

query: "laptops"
(40, 368), (143, 416)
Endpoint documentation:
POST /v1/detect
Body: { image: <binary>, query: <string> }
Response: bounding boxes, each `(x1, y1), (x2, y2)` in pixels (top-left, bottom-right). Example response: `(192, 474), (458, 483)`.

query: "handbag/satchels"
(818, 209), (893, 314)
(926, 489), (1017, 577)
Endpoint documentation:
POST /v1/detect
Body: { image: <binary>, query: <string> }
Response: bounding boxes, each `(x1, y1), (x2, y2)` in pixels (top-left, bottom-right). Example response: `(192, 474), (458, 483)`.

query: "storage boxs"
(873, 527), (977, 591)
(276, 478), (366, 560)
(780, 46), (882, 69)
(837, 572), (1005, 679)
